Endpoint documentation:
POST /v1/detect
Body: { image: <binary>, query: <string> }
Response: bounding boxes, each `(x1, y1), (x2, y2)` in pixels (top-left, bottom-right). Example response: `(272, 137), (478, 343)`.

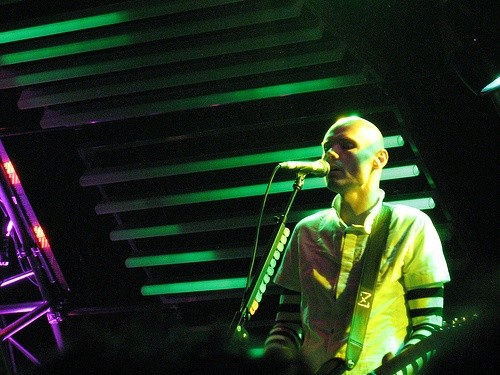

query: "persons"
(263, 116), (451, 375)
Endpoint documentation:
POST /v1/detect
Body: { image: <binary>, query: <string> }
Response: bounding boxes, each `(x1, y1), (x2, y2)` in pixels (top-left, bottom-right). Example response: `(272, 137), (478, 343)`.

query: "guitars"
(312, 312), (481, 375)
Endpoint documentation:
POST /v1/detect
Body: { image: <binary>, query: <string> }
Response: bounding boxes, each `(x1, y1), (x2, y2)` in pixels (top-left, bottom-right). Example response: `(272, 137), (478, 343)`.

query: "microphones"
(278, 159), (331, 177)
